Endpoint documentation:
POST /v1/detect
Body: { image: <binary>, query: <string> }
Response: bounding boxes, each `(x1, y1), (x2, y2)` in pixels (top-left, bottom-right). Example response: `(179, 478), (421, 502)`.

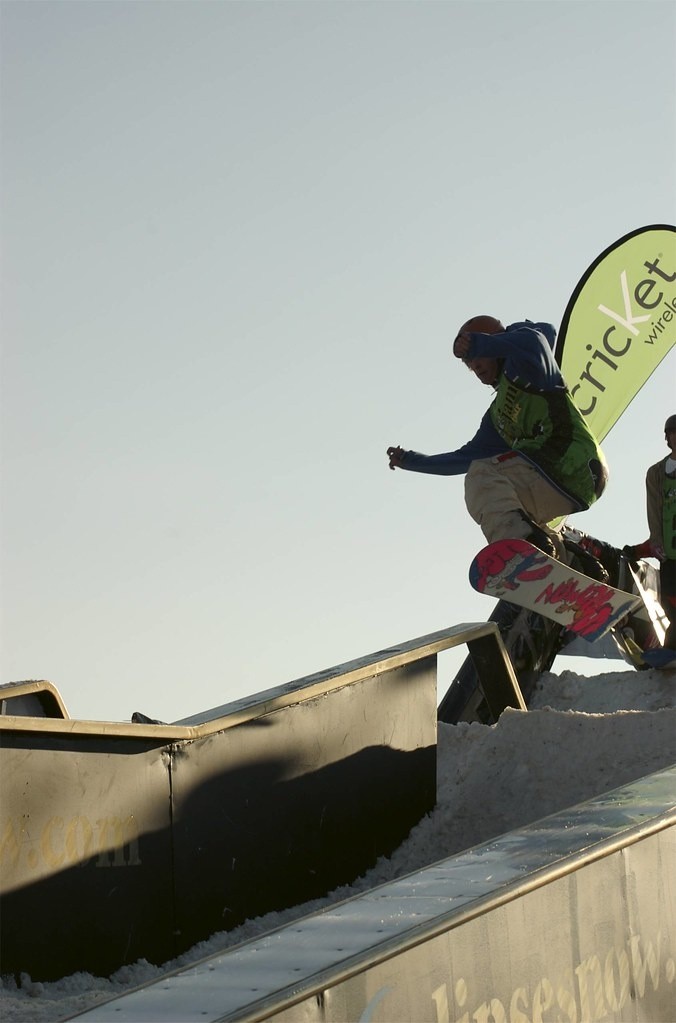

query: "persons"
(623, 414), (676, 652)
(387, 316), (609, 585)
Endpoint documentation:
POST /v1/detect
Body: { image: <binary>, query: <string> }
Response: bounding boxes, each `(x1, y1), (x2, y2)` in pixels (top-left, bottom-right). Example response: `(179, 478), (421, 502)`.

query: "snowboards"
(468, 538), (641, 644)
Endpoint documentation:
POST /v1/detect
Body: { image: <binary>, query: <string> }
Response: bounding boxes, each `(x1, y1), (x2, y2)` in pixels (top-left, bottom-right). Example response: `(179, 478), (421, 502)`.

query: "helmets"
(665, 415), (676, 431)
(459, 315), (505, 335)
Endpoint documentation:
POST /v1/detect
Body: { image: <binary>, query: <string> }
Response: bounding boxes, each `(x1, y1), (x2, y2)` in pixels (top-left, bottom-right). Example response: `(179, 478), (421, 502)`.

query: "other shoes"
(565, 540), (610, 584)
(527, 530), (557, 559)
